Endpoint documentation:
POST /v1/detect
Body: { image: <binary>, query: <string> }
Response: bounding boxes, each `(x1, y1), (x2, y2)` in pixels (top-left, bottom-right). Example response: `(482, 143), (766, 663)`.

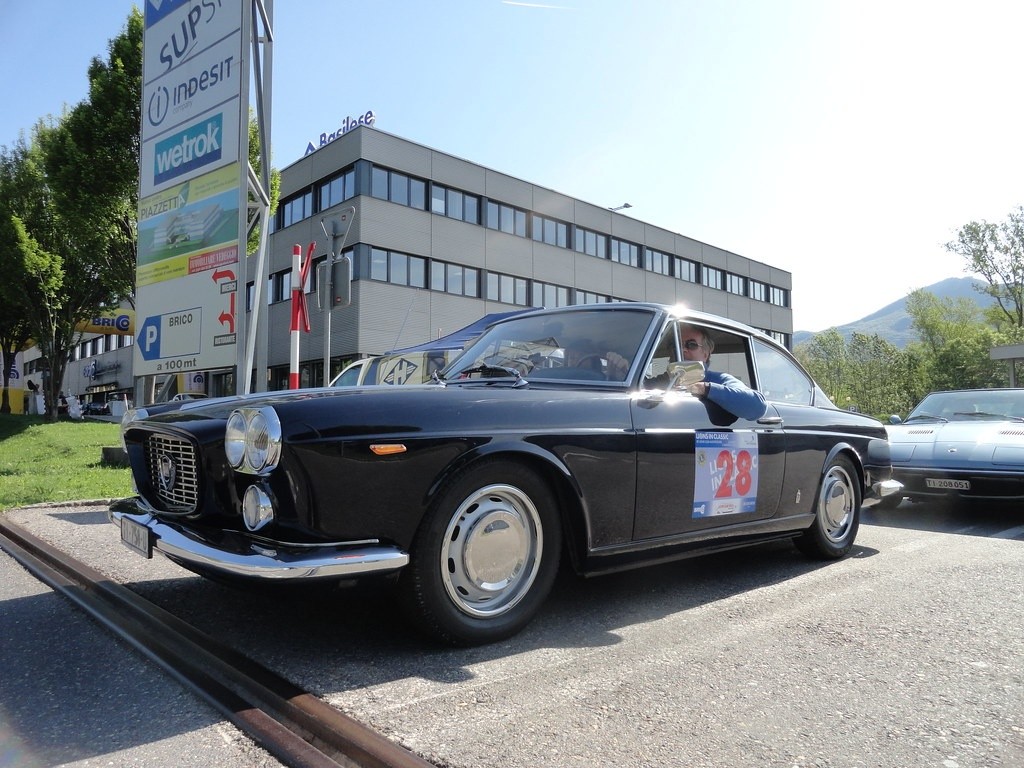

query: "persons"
(59, 391), (68, 405)
(81, 400), (85, 408)
(606, 324), (768, 421)
(562, 340), (600, 369)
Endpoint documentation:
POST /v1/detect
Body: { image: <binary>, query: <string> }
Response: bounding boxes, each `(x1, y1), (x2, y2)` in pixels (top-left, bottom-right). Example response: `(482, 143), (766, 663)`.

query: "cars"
(326, 352), (545, 389)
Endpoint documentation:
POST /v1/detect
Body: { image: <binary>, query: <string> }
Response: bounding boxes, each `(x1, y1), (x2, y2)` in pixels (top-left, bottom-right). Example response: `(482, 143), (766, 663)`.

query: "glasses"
(568, 353), (580, 358)
(667, 339), (703, 351)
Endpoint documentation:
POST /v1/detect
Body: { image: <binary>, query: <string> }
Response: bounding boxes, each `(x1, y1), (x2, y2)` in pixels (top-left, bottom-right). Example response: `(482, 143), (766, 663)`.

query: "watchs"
(703, 381), (711, 396)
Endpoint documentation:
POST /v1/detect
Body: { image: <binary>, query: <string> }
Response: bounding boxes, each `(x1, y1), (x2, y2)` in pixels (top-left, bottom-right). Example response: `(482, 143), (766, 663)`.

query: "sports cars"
(879, 386), (1024, 514)
(109, 303), (894, 646)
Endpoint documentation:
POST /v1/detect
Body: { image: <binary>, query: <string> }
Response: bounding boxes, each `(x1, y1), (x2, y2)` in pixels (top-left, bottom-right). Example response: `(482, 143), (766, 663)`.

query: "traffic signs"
(136, 304), (203, 362)
